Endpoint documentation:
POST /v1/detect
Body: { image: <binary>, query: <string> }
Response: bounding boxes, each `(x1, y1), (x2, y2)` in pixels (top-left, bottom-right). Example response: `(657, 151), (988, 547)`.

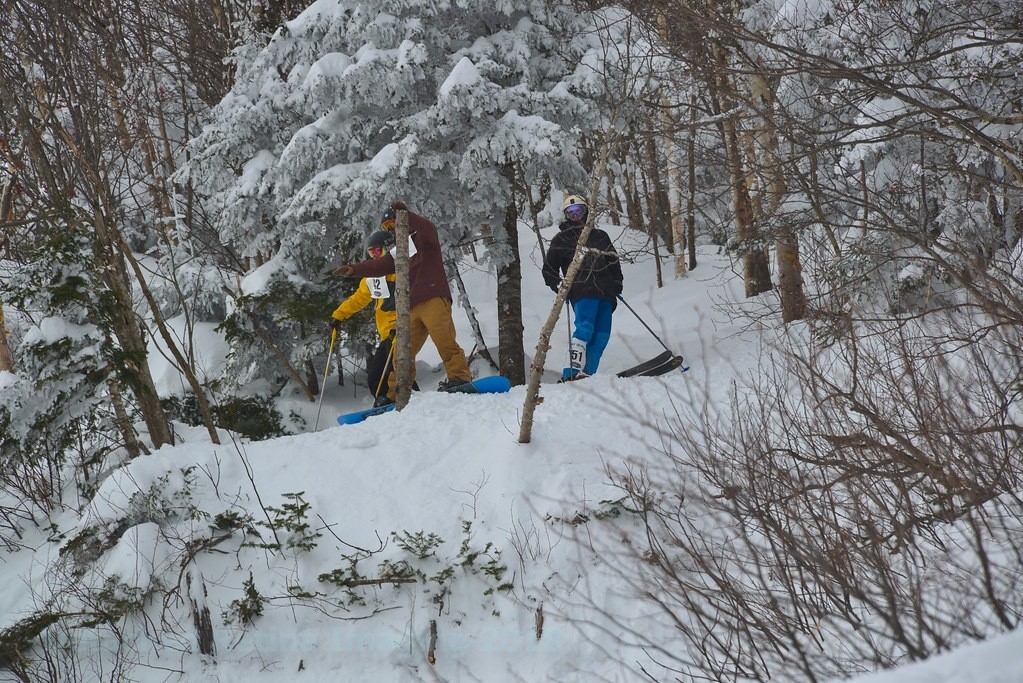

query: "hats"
(368, 230), (394, 248)
(379, 206), (398, 225)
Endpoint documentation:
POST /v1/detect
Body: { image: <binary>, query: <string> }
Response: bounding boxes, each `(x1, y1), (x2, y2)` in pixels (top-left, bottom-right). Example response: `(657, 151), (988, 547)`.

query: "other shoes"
(438, 375), (467, 392)
(561, 369), (589, 382)
(374, 395), (395, 407)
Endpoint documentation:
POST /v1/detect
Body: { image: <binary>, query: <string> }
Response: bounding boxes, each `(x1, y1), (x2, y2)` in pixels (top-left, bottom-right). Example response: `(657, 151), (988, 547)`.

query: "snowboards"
(336, 374), (511, 425)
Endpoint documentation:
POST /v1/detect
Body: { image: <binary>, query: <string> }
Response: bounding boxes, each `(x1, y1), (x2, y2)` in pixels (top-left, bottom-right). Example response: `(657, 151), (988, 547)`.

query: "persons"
(332, 201), (472, 407)
(542, 195), (623, 382)
(329, 230), (420, 405)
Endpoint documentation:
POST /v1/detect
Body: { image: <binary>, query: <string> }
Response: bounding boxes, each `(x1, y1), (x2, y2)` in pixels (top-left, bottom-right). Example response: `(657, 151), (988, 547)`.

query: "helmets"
(561, 194), (588, 212)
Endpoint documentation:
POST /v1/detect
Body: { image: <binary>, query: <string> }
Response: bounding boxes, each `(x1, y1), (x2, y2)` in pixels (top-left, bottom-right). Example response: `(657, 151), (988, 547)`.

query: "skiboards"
(611, 349), (687, 380)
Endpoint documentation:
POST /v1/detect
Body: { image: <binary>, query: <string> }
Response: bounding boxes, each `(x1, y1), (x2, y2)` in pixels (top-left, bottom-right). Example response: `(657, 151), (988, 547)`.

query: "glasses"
(381, 220), (395, 232)
(564, 205), (587, 218)
(367, 246), (387, 259)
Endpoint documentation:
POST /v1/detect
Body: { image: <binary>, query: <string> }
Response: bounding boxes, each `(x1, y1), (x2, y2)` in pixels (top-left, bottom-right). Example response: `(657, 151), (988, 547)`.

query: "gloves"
(329, 317), (341, 328)
(391, 201), (408, 212)
(614, 280), (623, 295)
(550, 278), (563, 293)
(333, 265), (356, 277)
